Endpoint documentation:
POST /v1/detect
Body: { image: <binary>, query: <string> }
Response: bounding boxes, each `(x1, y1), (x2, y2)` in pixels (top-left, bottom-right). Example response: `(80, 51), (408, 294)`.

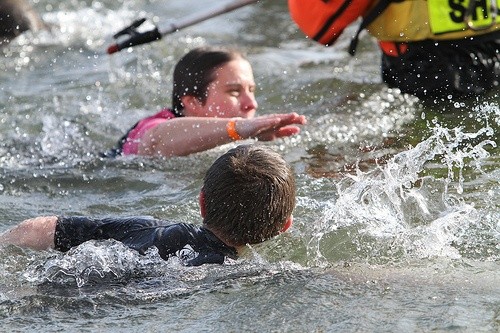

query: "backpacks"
(288, 1), (390, 56)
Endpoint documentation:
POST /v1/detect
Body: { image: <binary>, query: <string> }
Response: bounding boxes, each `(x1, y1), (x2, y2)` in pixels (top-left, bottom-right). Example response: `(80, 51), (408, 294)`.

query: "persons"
(361, 1), (499, 113)
(102, 46), (306, 160)
(1, 145), (296, 272)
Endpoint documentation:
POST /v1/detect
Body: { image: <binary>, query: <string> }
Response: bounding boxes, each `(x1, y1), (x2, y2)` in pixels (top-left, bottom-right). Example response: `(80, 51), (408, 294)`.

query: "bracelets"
(227, 117), (243, 141)
